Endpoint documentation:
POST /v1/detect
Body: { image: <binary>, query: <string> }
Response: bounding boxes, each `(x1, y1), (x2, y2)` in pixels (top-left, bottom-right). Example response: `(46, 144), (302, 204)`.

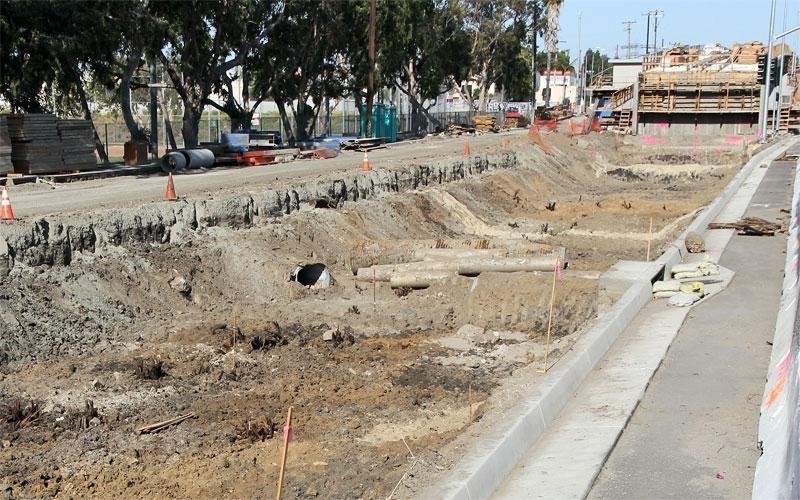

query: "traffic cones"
(1, 186), (14, 222)
(462, 140), (473, 161)
(162, 170), (181, 204)
(359, 146), (372, 172)
